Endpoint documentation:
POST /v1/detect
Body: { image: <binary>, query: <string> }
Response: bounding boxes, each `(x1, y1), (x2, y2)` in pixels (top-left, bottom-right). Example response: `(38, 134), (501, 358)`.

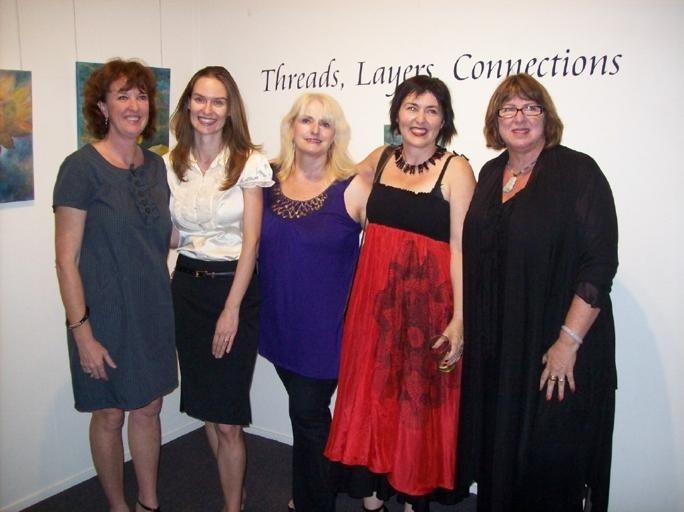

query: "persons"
(55, 60), (195, 511)
(460, 74), (622, 511)
(159, 67), (267, 511)
(245, 91), (378, 511)
(335, 76), (476, 512)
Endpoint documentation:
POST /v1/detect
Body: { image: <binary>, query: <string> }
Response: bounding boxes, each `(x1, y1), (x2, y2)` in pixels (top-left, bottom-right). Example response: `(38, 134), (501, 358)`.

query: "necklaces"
(395, 145), (448, 176)
(500, 160), (537, 193)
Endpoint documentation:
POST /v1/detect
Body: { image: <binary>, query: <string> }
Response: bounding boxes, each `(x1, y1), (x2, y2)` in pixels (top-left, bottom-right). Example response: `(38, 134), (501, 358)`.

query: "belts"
(174, 264), (236, 280)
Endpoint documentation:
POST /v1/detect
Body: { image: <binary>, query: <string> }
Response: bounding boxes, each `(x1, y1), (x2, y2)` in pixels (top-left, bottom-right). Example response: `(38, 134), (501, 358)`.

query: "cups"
(428, 334), (457, 374)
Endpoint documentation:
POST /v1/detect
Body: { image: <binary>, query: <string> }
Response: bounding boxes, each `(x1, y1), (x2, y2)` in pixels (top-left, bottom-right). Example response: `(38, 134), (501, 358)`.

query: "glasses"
(496, 106), (546, 119)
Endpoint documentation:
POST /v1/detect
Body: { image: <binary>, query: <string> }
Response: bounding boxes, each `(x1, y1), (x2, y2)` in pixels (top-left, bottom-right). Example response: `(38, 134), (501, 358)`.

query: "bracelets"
(66, 308), (91, 328)
(559, 322), (584, 350)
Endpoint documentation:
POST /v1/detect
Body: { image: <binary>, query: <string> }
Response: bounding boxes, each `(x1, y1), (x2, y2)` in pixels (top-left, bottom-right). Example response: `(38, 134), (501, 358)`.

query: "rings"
(547, 375), (566, 382)
(225, 338), (229, 343)
